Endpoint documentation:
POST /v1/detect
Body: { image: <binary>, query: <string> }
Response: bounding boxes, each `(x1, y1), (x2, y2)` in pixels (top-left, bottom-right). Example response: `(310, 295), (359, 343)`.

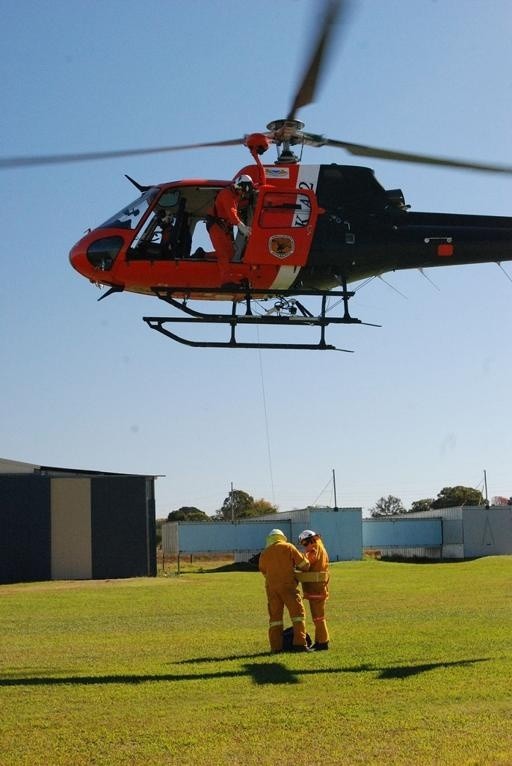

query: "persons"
(146, 214), (174, 250)
(296, 529), (330, 651)
(190, 174), (254, 263)
(258, 528), (310, 655)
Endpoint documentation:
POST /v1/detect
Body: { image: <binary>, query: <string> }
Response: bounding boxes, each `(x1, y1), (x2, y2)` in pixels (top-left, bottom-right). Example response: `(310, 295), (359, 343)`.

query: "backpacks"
(283, 626), (312, 653)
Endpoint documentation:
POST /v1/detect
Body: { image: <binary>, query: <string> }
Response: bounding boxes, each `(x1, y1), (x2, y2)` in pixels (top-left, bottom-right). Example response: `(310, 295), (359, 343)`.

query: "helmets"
(296, 529), (316, 544)
(268, 528), (285, 537)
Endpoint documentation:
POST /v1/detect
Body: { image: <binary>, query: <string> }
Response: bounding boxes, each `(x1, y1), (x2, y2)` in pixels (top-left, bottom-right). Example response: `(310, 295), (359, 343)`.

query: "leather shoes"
(272, 642), (328, 656)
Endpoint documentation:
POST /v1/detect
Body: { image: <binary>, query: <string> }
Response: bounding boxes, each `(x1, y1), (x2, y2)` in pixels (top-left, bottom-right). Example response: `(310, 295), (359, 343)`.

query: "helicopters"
(0, 1), (512, 352)
